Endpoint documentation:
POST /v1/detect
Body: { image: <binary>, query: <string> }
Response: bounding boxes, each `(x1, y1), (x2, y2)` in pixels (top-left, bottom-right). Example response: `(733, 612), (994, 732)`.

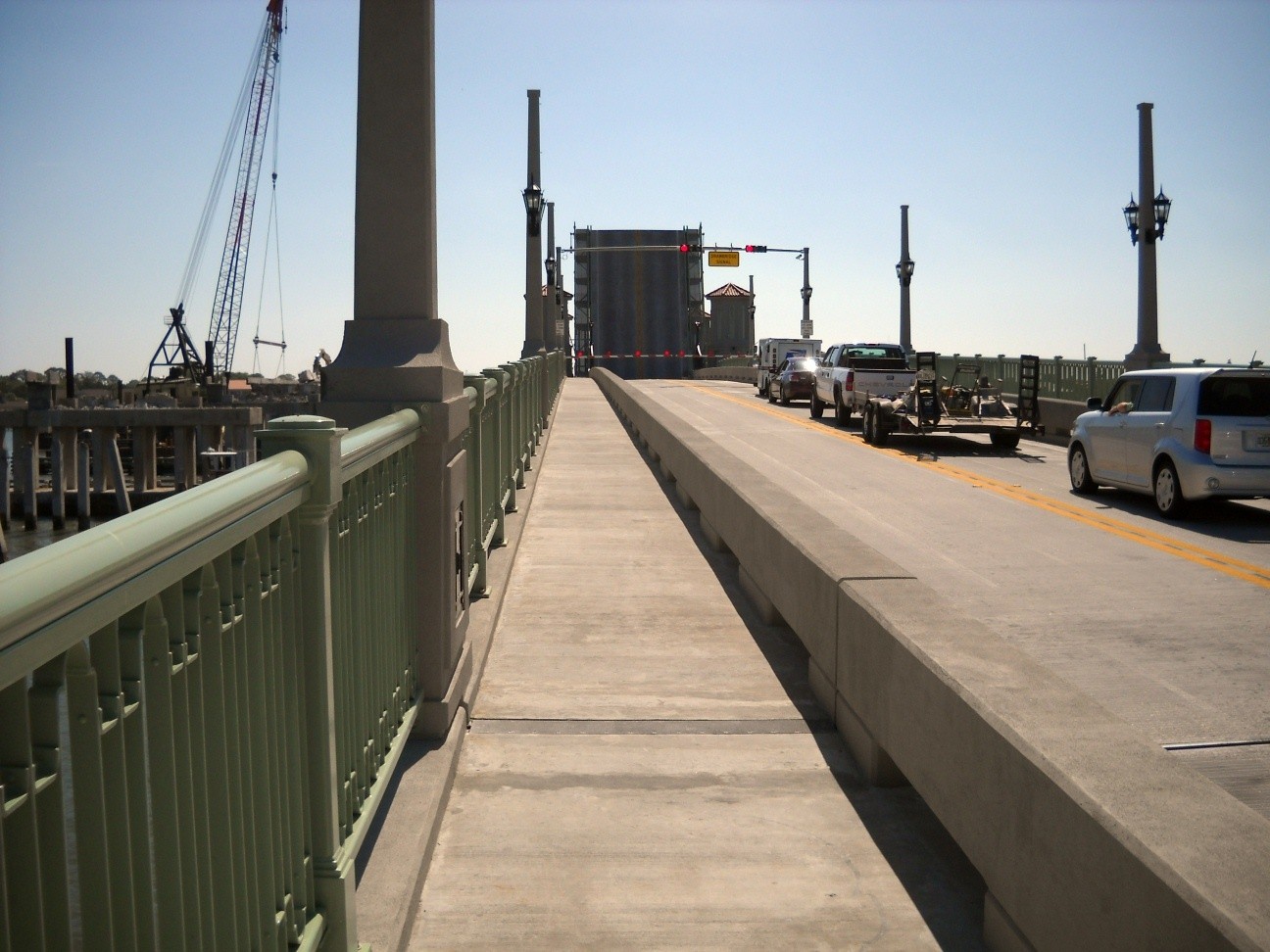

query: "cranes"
(141, 0), (292, 387)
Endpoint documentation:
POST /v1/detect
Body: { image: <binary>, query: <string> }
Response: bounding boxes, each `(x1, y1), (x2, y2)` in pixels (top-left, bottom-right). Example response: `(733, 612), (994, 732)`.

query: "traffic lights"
(745, 244), (767, 253)
(680, 244), (702, 252)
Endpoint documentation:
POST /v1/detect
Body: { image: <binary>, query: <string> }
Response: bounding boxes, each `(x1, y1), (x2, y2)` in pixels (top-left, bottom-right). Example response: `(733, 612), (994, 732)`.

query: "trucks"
(754, 338), (823, 398)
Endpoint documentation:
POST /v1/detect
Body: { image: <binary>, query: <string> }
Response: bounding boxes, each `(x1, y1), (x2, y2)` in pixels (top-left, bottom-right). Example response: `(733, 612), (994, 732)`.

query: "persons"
(1109, 402), (1133, 416)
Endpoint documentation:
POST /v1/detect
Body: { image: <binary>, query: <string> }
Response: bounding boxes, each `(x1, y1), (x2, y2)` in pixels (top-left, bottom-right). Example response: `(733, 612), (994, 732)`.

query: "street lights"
(521, 171), (569, 354)
(893, 204), (917, 345)
(1122, 101), (1171, 362)
(798, 248), (813, 336)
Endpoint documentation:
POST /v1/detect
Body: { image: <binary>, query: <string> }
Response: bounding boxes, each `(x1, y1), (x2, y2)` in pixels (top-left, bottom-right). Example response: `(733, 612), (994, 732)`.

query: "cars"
(769, 358), (825, 403)
(1066, 367), (1270, 518)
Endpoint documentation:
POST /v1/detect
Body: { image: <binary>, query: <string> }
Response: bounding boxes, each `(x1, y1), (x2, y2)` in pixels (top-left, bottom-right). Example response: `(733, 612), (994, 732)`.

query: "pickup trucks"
(809, 343), (930, 423)
